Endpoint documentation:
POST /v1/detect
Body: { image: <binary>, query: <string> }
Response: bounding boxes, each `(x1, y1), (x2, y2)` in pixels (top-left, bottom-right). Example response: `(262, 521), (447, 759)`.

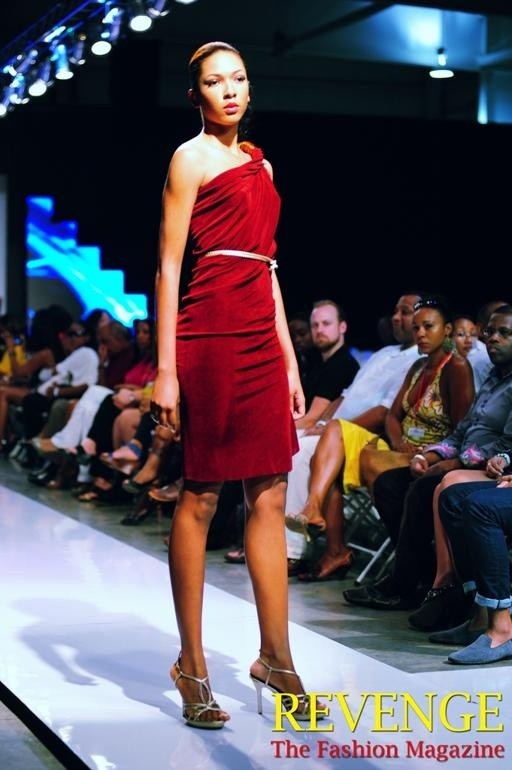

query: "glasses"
(64, 330), (85, 337)
(484, 329), (512, 337)
(412, 300), (437, 309)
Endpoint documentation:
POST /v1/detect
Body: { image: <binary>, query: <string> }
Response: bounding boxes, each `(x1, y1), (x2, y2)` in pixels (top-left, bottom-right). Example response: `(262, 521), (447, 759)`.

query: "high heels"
(1, 437), (247, 563)
(250, 658), (328, 721)
(170, 651), (225, 730)
(284, 512), (326, 542)
(297, 551), (354, 581)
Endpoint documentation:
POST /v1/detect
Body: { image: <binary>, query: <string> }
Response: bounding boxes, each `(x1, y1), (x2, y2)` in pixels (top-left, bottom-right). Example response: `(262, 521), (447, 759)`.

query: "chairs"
(312, 482), (396, 586)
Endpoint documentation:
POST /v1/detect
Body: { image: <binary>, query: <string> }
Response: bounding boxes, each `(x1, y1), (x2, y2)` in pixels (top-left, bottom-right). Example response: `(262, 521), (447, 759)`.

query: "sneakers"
(341, 573), (511, 665)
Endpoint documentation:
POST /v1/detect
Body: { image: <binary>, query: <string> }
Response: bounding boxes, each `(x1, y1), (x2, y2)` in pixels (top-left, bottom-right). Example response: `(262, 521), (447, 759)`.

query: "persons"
(148, 39), (330, 732)
(3, 286), (512, 667)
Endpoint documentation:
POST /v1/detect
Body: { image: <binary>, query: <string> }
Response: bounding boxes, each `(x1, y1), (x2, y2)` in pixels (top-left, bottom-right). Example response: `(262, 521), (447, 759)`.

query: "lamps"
(429, 10), (454, 78)
(0, 0), (168, 116)
